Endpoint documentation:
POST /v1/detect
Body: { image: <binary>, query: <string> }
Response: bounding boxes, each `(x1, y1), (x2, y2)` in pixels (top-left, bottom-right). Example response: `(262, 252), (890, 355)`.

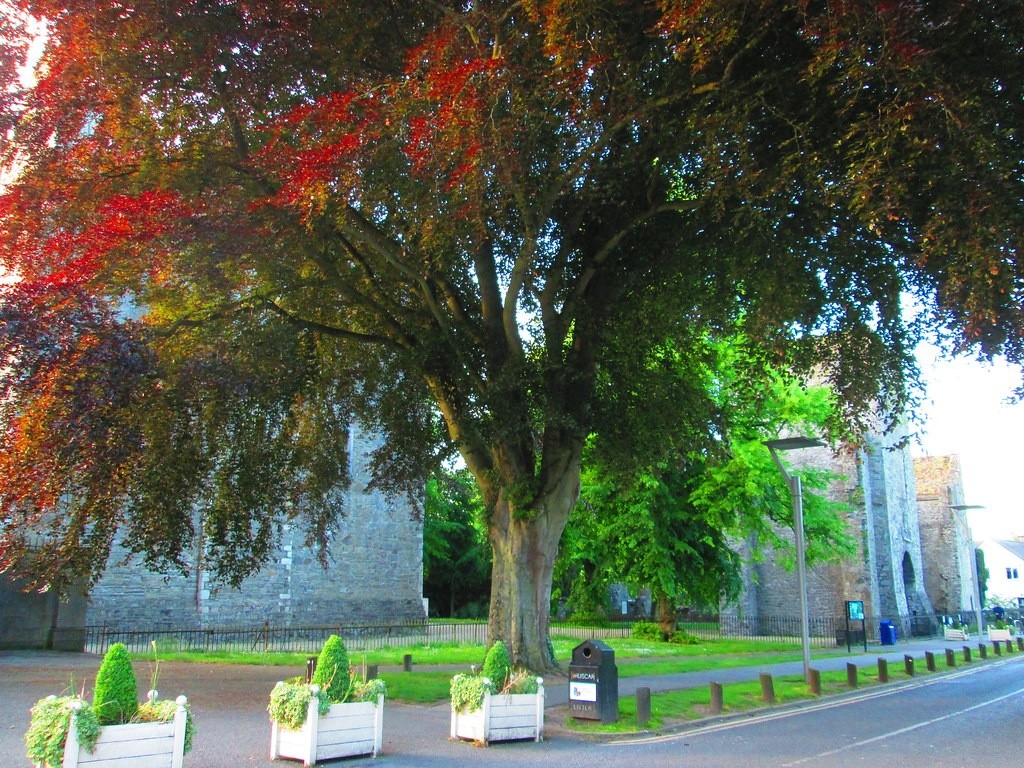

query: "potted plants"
(450, 639), (545, 748)
(269, 633), (386, 768)
(24, 640), (194, 768)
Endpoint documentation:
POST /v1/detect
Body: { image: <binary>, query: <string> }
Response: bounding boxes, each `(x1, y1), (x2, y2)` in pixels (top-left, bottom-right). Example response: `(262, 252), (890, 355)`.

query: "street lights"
(759, 435), (828, 685)
(949, 504), (985, 643)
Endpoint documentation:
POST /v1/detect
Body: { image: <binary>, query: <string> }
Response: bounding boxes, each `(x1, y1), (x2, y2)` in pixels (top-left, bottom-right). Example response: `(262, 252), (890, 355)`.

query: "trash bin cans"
(880, 620), (896, 645)
(568, 639), (619, 726)
